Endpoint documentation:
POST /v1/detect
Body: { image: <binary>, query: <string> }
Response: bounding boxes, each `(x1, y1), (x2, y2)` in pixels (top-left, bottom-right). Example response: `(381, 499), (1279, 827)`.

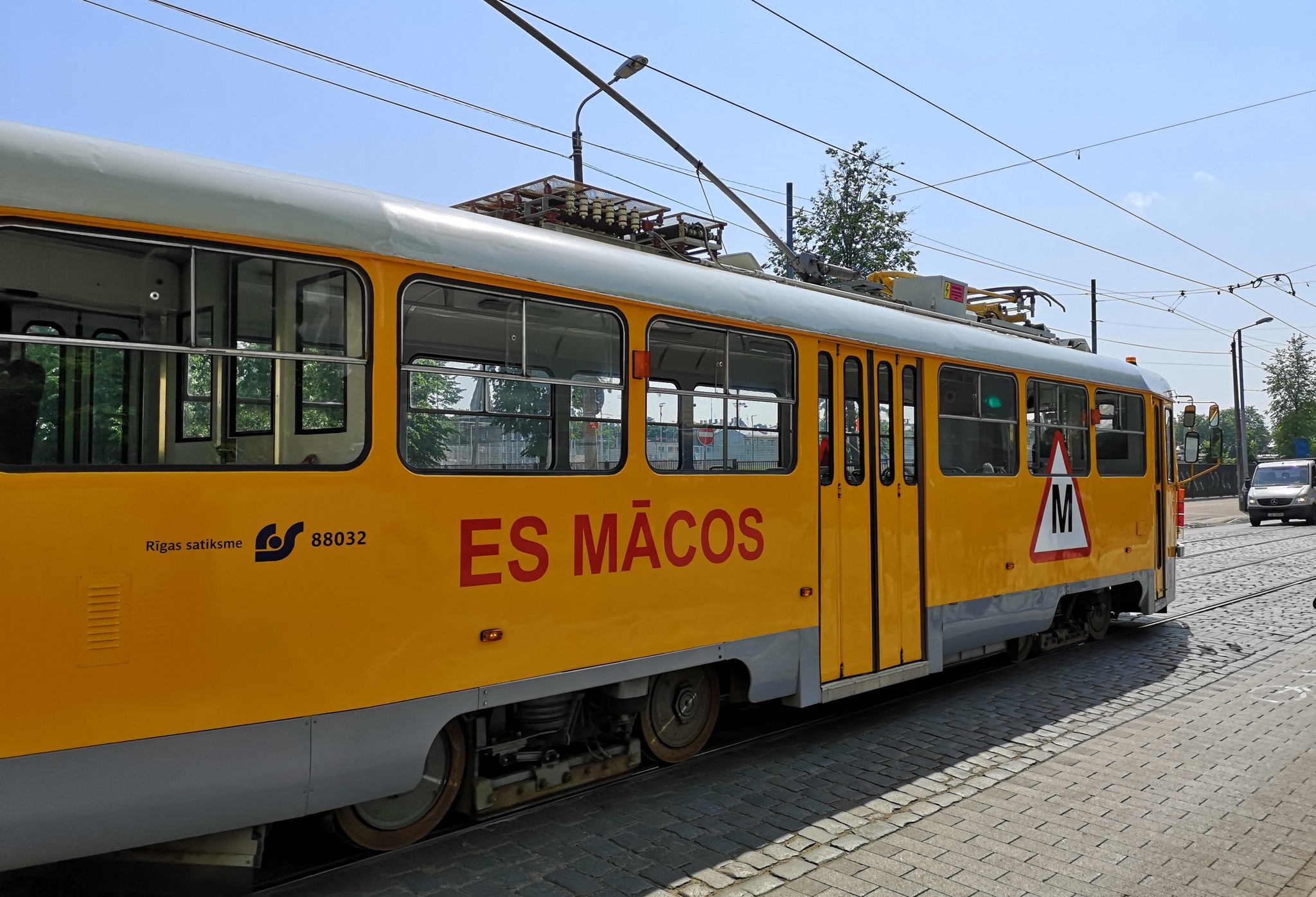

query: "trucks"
(1247, 458), (1316, 526)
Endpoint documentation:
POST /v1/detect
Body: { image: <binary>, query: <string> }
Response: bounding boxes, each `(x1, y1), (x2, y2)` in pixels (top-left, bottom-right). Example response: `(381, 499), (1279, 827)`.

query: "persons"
(1031, 427), (1067, 473)
(1299, 470), (1315, 483)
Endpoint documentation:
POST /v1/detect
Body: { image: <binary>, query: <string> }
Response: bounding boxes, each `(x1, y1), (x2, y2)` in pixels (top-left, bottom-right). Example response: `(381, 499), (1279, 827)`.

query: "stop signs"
(697, 423), (715, 446)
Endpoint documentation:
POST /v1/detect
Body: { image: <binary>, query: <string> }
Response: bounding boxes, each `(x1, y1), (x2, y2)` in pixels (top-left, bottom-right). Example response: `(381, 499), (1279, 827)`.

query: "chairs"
(817, 463), (1007, 485)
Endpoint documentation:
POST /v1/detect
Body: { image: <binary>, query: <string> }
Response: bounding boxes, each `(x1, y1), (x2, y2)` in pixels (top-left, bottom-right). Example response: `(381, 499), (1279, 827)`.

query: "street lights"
(572, 55), (649, 193)
(752, 415), (758, 461)
(659, 402), (666, 458)
(1231, 317), (1274, 511)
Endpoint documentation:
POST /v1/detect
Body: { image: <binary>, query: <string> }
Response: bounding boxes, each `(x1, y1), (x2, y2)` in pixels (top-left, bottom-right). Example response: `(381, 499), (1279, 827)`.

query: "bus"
(0, 123), (1223, 871)
